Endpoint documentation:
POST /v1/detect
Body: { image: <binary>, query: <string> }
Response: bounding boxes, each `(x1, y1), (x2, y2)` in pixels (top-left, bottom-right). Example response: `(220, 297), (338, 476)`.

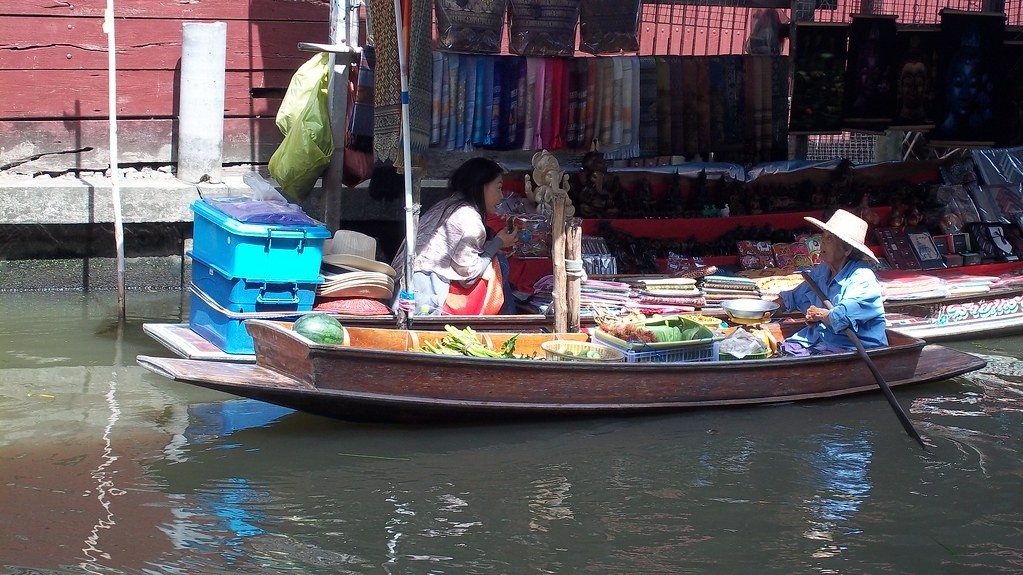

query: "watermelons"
(291, 312), (344, 345)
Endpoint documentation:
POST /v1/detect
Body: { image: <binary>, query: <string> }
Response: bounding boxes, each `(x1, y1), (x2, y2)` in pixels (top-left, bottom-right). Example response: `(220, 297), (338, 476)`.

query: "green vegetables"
(408, 323), (542, 361)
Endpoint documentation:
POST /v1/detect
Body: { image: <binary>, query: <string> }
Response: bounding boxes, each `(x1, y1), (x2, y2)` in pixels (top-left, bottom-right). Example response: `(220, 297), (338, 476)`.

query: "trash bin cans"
(720, 299), (781, 358)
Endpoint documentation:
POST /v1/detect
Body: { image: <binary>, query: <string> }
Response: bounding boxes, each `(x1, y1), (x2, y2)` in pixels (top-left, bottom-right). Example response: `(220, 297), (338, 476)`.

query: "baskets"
(719, 345), (769, 361)
(592, 318), (719, 364)
(806, 131), (883, 161)
(542, 341), (626, 363)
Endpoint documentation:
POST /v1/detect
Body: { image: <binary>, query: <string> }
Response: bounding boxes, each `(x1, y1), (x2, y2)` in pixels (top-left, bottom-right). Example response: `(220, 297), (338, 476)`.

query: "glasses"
(816, 230), (848, 248)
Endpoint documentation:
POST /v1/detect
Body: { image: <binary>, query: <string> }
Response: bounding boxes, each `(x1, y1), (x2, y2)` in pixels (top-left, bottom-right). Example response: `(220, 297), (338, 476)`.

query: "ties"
(876, 221), (1023, 273)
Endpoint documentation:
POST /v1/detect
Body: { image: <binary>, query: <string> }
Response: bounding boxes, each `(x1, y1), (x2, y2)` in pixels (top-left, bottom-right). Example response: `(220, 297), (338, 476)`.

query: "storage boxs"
(588, 333), (720, 363)
(190, 199), (332, 280)
(932, 235), (963, 267)
(951, 233), (981, 266)
(186, 250), (325, 356)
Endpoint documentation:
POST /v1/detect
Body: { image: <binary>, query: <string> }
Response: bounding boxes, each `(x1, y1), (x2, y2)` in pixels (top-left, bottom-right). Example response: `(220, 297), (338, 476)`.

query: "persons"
(772, 208), (890, 356)
(529, 151), (576, 217)
(385, 154), (520, 316)
(576, 153), (616, 210)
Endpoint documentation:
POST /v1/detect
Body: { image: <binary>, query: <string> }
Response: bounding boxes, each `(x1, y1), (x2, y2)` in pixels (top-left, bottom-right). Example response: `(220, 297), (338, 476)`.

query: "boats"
(135, 314), (988, 425)
(146, 260), (1023, 359)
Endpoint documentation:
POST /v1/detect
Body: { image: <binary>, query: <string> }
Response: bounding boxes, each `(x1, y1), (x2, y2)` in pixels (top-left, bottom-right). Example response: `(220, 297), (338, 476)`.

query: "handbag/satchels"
(268, 61), (334, 202)
(274, 50), (329, 135)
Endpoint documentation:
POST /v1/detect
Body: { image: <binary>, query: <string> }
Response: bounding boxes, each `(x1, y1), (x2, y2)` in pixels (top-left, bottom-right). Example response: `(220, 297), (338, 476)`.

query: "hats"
(315, 229), (395, 315)
(803, 209), (881, 264)
